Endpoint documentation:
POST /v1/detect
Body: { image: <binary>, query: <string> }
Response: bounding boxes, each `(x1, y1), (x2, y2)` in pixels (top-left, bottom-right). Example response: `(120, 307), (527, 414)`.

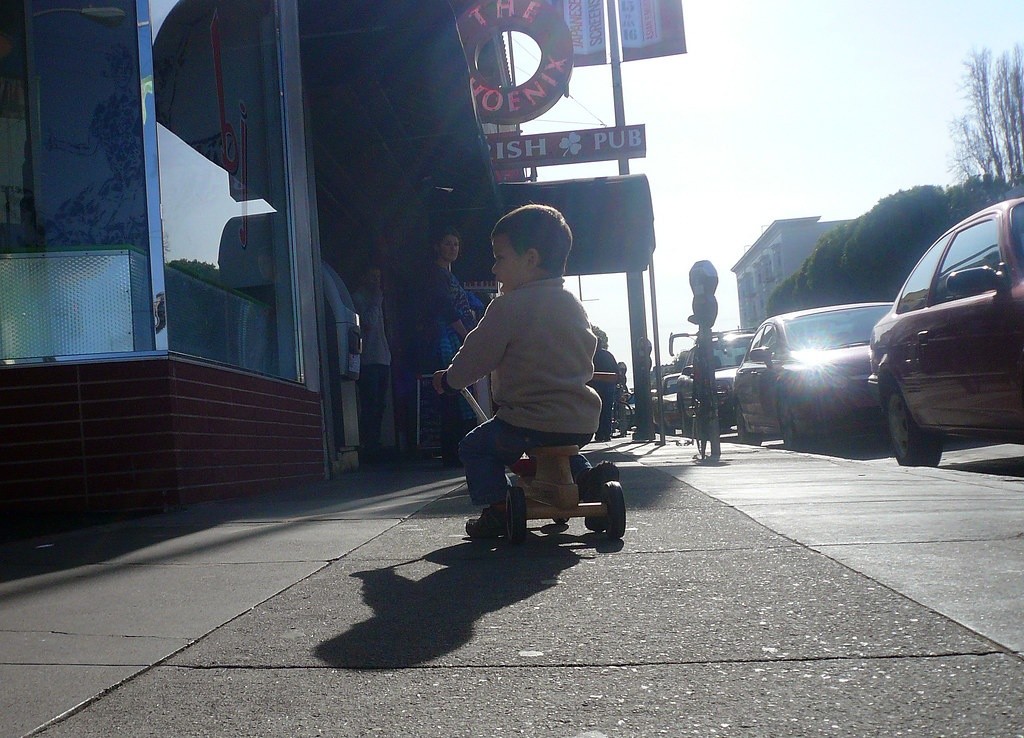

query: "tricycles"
(420, 368), (627, 546)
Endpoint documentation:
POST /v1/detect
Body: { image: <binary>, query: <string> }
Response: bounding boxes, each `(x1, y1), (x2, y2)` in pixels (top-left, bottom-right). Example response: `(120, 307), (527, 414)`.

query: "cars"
(651, 373), (682, 435)
(866, 197), (1024, 468)
(611, 392), (636, 429)
(677, 334), (755, 438)
(731, 301), (897, 452)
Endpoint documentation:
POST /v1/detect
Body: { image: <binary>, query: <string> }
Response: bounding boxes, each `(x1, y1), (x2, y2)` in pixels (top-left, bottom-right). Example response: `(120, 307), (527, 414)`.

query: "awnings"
(300, 0), (503, 252)
(153, 0), (288, 218)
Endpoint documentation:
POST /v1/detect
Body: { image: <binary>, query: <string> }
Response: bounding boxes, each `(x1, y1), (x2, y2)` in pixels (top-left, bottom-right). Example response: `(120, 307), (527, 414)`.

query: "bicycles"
(669, 332), (726, 462)
(610, 392), (633, 438)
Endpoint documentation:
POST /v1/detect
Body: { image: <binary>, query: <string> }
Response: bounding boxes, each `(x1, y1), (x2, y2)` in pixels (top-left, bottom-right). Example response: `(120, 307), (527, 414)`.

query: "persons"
(590, 337), (618, 442)
(347, 225), (477, 469)
(431, 205), (619, 539)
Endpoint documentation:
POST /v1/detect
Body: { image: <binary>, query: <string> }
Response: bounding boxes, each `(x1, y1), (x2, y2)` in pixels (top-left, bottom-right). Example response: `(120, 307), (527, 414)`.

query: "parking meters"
(690, 259), (721, 461)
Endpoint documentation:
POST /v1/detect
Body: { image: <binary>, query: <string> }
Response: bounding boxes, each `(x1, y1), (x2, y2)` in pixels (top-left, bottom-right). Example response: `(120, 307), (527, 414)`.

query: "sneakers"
(581, 461), (621, 532)
(465, 508), (508, 538)
